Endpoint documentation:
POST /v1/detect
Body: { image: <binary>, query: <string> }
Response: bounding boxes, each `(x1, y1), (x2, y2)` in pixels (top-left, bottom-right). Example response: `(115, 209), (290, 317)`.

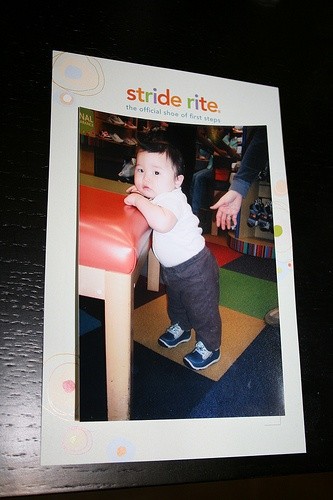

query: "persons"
(124, 139), (222, 370)
(191, 125), (242, 224)
(210, 125), (268, 230)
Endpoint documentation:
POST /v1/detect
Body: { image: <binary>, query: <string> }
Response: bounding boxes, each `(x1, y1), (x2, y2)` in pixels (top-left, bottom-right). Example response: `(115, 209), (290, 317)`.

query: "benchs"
(77, 173), (160, 421)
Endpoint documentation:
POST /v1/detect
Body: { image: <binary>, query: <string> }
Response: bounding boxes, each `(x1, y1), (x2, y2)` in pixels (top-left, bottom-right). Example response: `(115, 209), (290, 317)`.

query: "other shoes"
(264, 308), (280, 328)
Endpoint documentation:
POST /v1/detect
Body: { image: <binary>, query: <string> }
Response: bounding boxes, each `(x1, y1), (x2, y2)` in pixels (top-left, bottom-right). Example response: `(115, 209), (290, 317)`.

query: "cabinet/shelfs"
(94, 111), (138, 184)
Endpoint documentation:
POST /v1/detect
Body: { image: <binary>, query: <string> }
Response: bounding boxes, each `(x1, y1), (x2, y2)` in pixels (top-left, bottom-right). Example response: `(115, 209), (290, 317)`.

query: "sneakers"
(157, 323), (193, 349)
(183, 341), (222, 371)
